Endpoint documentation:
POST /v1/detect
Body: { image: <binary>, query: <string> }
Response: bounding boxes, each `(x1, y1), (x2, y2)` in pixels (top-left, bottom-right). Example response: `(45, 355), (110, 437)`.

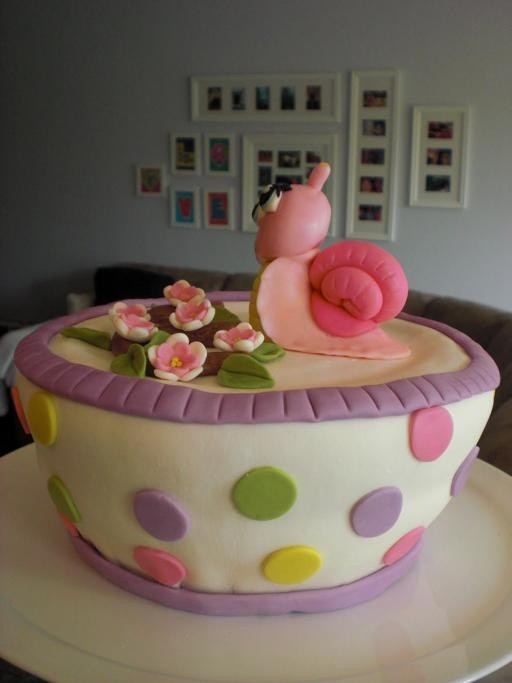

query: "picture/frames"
(129, 67), (474, 251)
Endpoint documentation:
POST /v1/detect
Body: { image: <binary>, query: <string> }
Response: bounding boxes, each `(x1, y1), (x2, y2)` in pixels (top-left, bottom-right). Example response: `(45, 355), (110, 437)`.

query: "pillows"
(91, 264), (175, 307)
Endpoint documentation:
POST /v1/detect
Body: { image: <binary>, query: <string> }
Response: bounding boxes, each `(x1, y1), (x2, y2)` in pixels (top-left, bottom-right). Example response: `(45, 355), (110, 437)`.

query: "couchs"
(64, 254), (510, 471)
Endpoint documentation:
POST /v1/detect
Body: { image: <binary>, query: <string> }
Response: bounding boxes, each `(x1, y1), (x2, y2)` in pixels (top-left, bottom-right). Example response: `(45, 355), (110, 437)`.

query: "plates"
(1, 441), (512, 681)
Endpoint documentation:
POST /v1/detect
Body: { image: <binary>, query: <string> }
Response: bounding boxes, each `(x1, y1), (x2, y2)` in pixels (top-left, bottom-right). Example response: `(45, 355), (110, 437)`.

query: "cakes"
(5, 160), (502, 619)
